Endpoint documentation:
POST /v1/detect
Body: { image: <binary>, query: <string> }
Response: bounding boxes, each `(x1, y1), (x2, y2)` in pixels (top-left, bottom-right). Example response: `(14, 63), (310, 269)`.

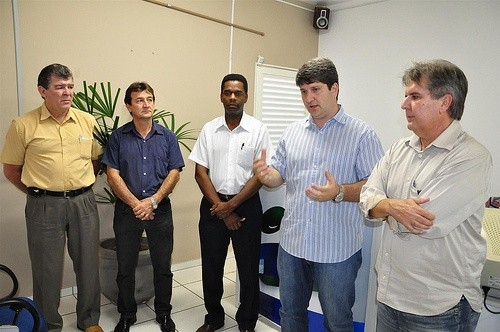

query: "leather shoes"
(195, 321), (225, 332)
(238, 322), (256, 332)
(76, 322), (104, 332)
(114, 313), (137, 332)
(156, 314), (176, 332)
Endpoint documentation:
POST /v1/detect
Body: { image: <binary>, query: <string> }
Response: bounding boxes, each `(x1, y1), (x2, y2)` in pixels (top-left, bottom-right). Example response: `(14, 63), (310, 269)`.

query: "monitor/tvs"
(481, 208), (500, 298)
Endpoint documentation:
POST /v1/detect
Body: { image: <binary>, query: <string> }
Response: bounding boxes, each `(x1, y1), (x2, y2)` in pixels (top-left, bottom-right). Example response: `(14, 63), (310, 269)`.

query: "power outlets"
(487, 275), (500, 289)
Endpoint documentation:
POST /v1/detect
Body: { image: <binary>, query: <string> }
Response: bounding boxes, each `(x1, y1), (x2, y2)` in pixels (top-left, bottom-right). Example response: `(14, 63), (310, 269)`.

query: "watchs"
(150, 197), (158, 210)
(331, 184), (344, 203)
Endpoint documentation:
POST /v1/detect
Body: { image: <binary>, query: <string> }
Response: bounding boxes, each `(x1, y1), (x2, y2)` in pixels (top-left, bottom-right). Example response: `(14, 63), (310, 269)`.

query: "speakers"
(313, 7), (330, 29)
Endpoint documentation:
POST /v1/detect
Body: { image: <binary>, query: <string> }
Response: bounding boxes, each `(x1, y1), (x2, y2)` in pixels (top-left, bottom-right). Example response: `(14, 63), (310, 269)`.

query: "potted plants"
(71, 81), (202, 306)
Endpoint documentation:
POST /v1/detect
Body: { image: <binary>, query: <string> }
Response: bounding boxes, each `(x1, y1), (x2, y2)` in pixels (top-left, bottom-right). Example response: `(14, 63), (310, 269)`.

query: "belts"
(28, 185), (93, 198)
(218, 194), (236, 200)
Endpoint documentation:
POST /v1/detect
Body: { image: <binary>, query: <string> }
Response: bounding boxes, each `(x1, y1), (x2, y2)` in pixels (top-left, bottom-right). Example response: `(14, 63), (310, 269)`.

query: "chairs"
(0, 264), (48, 332)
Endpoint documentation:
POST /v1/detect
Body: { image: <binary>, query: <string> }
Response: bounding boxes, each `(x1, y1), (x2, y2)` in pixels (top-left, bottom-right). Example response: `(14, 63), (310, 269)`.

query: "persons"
(252, 56), (385, 332)
(359, 59), (494, 332)
(101, 81), (186, 332)
(186, 73), (274, 332)
(0, 64), (105, 332)
